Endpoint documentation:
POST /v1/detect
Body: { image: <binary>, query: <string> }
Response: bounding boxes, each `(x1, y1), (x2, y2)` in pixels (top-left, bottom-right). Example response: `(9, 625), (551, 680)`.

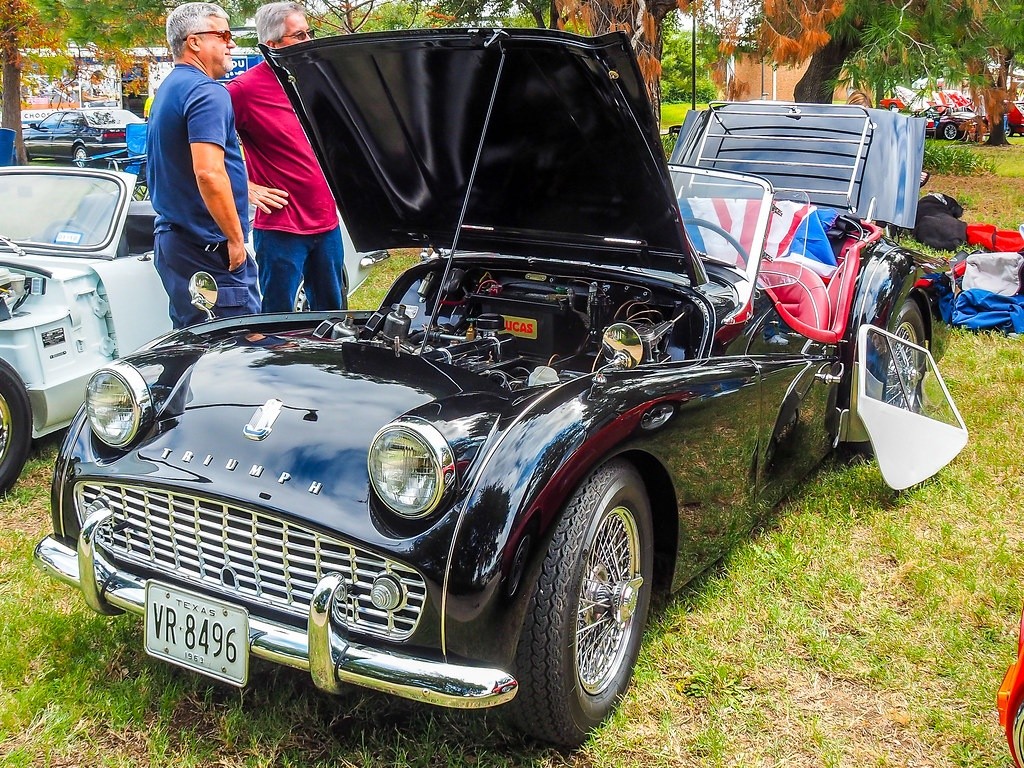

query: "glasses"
(282, 28), (315, 41)
(182, 29), (233, 44)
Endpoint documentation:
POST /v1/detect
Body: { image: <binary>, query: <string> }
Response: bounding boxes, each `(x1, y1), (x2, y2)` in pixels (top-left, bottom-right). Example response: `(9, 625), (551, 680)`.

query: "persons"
(80, 70), (109, 106)
(226, 2), (344, 314)
(144, 88), (158, 122)
(147, 2), (261, 328)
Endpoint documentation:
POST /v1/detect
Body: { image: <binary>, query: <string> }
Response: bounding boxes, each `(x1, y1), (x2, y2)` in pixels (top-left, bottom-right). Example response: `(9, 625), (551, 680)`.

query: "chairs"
(740, 259), (831, 330)
(77, 193), (130, 257)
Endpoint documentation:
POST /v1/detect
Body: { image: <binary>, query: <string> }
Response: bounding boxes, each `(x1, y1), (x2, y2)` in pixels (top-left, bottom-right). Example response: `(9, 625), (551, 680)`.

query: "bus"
(0, 41), (266, 130)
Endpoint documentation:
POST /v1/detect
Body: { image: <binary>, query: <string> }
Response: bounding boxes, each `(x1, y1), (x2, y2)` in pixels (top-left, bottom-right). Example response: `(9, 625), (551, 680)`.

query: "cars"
(0, 164), (390, 497)
(880, 86), (930, 113)
(34, 23), (934, 748)
(911, 105), (982, 141)
(14, 107), (146, 168)
(928, 90), (1024, 136)
